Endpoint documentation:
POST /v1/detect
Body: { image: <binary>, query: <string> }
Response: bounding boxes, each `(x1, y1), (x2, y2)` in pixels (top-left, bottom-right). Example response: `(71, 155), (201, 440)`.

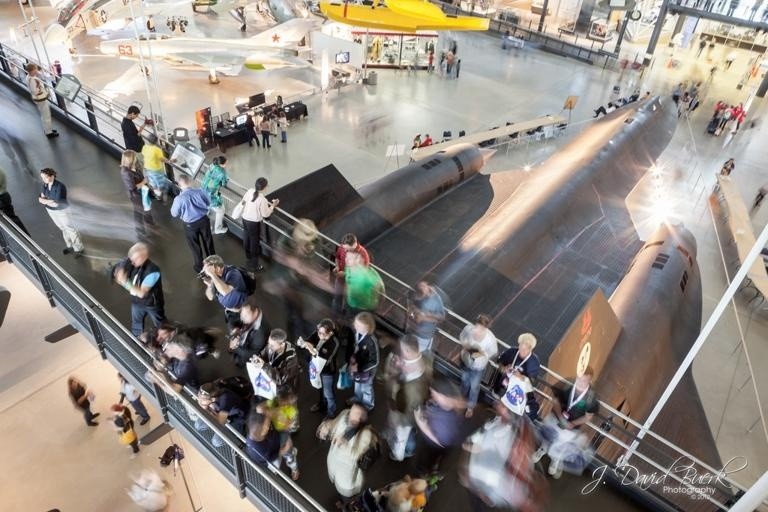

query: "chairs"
(409, 114), (566, 163)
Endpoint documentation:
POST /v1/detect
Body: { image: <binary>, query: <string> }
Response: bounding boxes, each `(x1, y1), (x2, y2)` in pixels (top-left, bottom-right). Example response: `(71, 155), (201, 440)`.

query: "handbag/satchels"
(501, 375), (534, 417)
(245, 362), (277, 400)
(337, 364), (352, 390)
(119, 430), (136, 445)
(309, 356), (328, 390)
(231, 200), (245, 219)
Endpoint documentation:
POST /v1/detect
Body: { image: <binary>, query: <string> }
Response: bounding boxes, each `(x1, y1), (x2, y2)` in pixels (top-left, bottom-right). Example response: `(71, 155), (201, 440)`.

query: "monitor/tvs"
(249, 92), (266, 108)
(336, 51), (350, 64)
(236, 113), (248, 127)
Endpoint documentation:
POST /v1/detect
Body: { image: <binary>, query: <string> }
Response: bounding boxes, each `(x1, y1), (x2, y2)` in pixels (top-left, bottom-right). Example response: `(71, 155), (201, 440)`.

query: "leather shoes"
(89, 413), (99, 426)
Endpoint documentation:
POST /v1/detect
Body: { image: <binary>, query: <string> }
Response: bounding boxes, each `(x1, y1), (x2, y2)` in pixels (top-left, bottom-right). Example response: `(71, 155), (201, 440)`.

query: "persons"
(0, 15), (768, 512)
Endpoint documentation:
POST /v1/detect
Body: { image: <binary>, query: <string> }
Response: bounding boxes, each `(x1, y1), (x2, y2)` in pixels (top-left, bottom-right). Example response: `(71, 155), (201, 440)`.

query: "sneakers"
(63, 247), (84, 260)
(215, 227), (228, 235)
(532, 446), (546, 463)
(548, 456), (559, 475)
(140, 416), (149, 424)
(44, 129), (59, 138)
(286, 447), (299, 480)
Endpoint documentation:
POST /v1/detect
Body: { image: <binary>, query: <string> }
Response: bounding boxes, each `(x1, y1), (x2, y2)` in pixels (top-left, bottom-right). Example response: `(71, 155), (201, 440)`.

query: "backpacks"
(213, 376), (254, 400)
(222, 264), (256, 296)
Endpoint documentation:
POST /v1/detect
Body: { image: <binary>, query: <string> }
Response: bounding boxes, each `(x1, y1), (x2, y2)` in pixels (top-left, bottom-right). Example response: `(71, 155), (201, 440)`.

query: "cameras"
(196, 268), (210, 279)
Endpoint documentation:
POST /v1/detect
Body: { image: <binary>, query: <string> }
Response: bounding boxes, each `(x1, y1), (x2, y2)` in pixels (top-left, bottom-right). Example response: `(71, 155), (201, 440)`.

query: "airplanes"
(211, 0), (322, 32)
(307, 0), (492, 37)
(99, 17), (319, 96)
(266, 89), (735, 512)
(40, 0), (141, 55)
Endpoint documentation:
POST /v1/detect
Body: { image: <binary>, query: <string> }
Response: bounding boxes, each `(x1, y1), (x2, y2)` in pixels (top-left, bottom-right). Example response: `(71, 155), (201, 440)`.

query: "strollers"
(347, 471), (445, 511)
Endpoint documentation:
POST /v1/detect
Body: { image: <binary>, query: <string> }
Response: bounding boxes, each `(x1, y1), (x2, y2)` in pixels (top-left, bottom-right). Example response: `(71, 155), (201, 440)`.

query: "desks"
(713, 174), (768, 311)
(506, 36), (524, 49)
(213, 93), (309, 154)
